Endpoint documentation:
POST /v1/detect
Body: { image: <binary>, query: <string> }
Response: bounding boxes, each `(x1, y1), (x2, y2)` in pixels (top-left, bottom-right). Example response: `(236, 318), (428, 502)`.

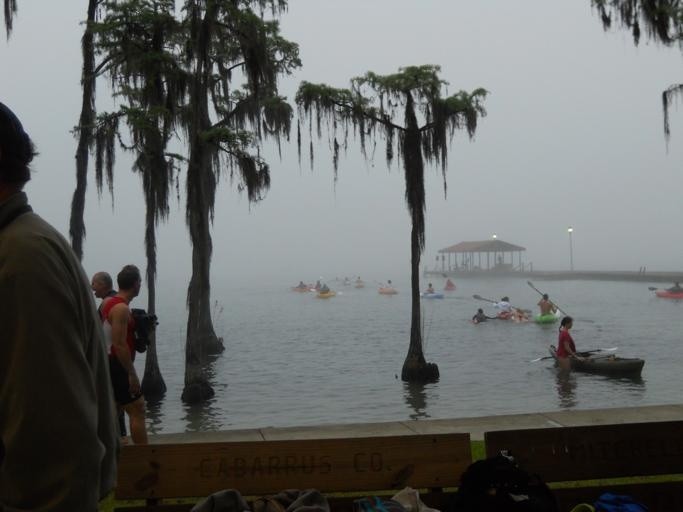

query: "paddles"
(472, 294), (526, 314)
(526, 281), (567, 316)
(648, 287), (669, 290)
(531, 347), (617, 362)
(310, 289), (343, 295)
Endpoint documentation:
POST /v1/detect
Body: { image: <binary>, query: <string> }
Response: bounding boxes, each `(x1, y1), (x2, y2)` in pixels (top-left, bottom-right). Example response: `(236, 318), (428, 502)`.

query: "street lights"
(567, 227), (572, 271)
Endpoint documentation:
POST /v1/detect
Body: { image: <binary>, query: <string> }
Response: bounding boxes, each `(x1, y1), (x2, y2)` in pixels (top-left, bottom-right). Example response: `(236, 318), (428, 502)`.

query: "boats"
(292, 284), (334, 298)
(421, 292), (445, 298)
(377, 286), (399, 294)
(548, 344), (644, 376)
(355, 283), (363, 289)
(535, 307), (560, 323)
(655, 289), (683, 298)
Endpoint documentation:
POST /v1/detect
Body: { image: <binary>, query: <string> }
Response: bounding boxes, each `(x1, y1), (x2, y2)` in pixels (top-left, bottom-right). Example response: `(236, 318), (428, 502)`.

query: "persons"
(90, 271), (127, 437)
(537, 293), (557, 315)
(492, 296), (513, 313)
(425, 283), (434, 293)
(99, 264), (150, 443)
(472, 308), (496, 321)
(0, 104), (119, 511)
(319, 283), (329, 293)
(663, 281), (683, 293)
(296, 281), (306, 288)
(355, 276), (361, 282)
(312, 280), (321, 288)
(555, 316), (577, 371)
(445, 278), (454, 288)
(384, 279), (392, 288)
